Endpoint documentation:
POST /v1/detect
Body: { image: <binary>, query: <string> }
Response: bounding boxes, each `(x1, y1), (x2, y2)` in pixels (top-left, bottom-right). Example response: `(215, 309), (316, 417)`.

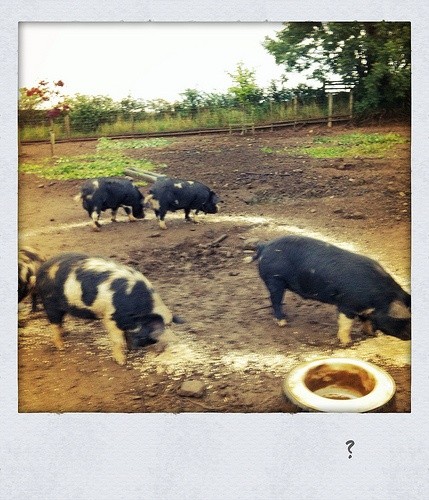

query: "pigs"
(246, 235), (411, 346)
(143, 177), (221, 229)
(35, 252), (187, 367)
(18, 244), (49, 328)
(73, 177), (151, 231)
